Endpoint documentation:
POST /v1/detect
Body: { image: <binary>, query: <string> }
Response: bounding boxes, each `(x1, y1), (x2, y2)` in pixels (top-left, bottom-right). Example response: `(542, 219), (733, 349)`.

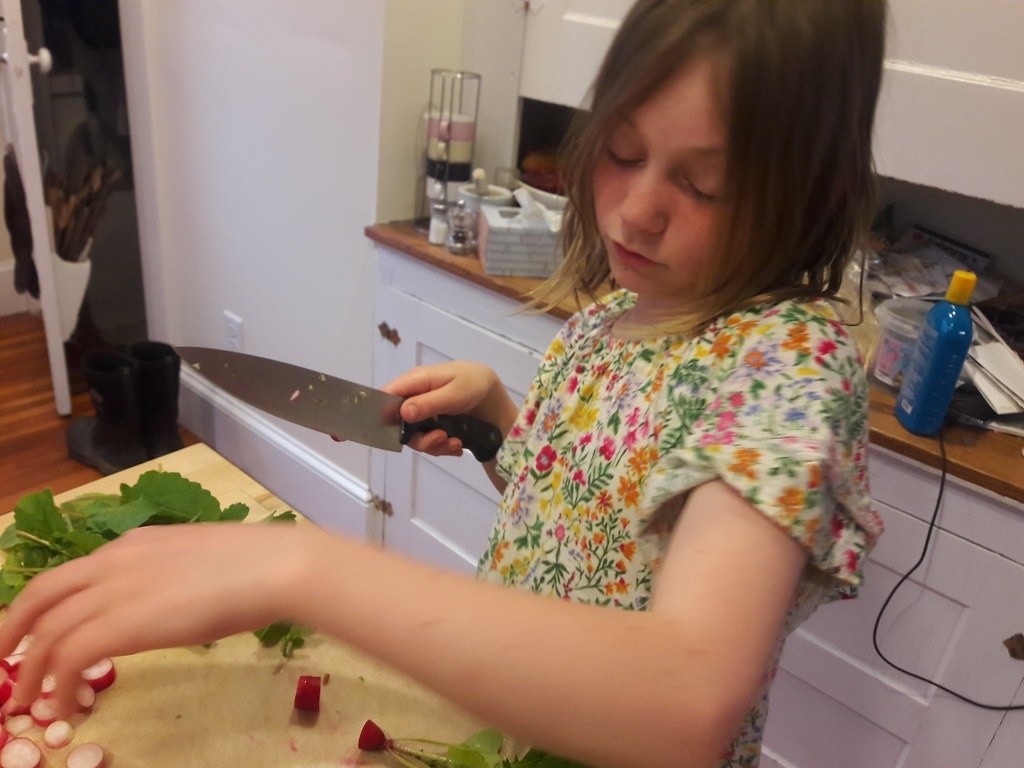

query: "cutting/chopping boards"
(0, 441), (536, 768)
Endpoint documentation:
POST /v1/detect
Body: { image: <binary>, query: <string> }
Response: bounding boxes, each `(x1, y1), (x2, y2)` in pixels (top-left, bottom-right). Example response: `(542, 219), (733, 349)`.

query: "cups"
(458, 184), (516, 205)
(863, 297), (939, 416)
(425, 112), (476, 228)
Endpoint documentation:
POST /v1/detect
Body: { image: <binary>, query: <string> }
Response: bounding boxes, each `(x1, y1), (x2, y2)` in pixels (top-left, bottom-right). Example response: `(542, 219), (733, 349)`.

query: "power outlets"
(221, 310), (242, 350)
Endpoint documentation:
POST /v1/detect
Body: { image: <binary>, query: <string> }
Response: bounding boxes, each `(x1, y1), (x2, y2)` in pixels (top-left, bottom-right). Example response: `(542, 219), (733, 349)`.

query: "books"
(964, 291), (1024, 415)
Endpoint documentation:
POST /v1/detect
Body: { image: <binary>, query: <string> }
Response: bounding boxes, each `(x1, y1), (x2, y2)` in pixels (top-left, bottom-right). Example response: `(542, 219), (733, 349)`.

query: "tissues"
(477, 186), (614, 282)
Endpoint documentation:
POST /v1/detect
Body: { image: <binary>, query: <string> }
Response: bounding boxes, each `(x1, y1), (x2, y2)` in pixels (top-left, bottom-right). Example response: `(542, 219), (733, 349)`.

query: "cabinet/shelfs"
(365, 242), (1024, 768)
(522, 1), (1023, 211)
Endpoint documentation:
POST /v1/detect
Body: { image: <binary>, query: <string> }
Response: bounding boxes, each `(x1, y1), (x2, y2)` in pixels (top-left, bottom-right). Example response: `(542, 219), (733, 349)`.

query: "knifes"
(172, 344), (504, 461)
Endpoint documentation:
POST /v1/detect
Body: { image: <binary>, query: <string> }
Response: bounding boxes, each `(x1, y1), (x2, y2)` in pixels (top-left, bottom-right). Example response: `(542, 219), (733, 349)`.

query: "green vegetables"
(0, 468), (317, 657)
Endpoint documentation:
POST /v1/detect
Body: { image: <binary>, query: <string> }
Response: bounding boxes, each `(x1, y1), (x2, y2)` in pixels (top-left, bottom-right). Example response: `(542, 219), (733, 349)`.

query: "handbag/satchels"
(45, 122), (124, 260)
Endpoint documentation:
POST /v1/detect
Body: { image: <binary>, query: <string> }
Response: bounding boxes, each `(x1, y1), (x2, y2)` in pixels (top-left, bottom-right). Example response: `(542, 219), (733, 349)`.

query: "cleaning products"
(894, 268), (978, 438)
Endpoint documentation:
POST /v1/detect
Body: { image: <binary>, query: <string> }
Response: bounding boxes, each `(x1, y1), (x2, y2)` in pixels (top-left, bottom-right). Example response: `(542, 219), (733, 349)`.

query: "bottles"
(894, 269), (978, 438)
(428, 195), (477, 256)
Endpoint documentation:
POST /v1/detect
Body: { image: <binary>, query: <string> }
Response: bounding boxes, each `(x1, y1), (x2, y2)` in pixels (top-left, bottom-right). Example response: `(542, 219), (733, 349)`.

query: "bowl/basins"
(509, 170), (572, 211)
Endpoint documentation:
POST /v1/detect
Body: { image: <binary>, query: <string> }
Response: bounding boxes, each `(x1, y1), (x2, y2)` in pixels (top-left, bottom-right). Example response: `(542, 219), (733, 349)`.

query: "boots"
(121, 343), (179, 459)
(66, 342), (147, 476)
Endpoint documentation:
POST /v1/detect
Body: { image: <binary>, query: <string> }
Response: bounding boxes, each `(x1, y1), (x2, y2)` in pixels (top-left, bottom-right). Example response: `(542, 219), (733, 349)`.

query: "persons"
(0, 0), (887, 767)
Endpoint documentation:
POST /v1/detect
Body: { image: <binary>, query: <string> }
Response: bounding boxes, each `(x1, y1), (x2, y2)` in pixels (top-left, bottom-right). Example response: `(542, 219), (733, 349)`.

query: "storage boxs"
(477, 205), (567, 277)
